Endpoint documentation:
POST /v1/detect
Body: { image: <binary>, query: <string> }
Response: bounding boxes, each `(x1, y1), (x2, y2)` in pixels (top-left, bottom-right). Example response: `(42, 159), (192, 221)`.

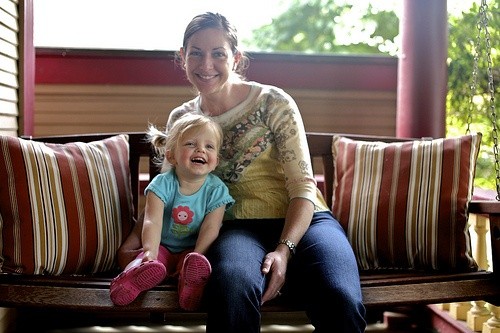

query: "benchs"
(0, 131), (500, 311)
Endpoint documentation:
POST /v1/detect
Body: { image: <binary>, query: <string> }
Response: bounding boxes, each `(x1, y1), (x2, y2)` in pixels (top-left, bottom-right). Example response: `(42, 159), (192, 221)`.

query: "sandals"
(110, 260), (166, 306)
(178, 252), (212, 310)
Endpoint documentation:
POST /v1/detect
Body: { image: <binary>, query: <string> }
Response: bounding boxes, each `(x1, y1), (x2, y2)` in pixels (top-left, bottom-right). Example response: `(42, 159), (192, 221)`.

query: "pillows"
(0, 133), (138, 278)
(331, 133), (483, 272)
